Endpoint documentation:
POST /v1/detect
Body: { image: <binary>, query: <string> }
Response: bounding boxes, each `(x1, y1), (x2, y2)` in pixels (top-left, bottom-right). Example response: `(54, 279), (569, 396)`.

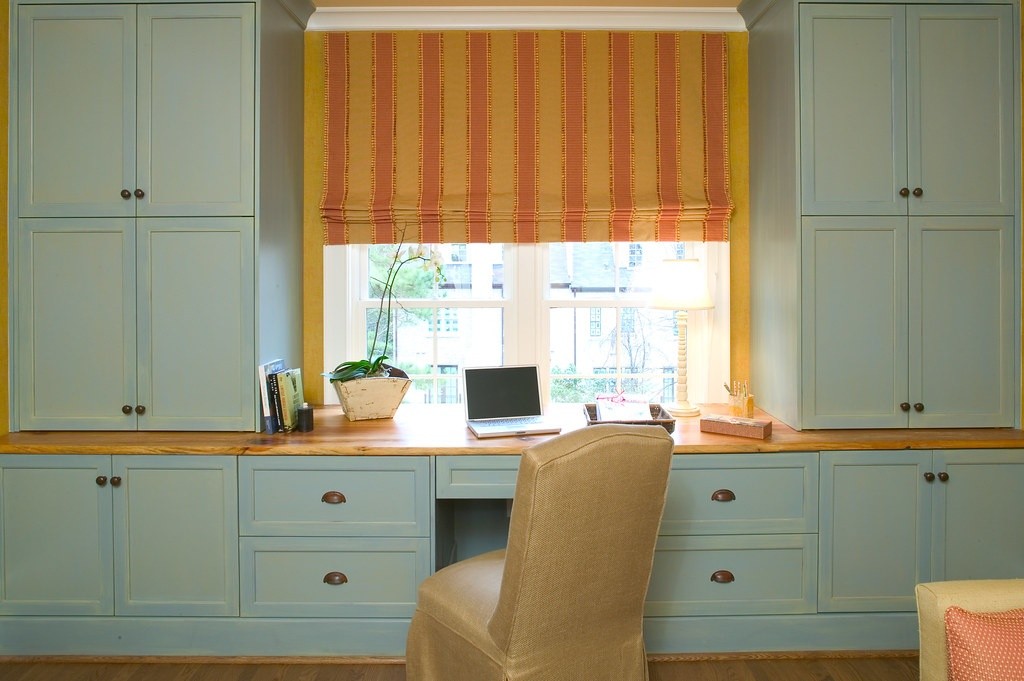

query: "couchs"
(914, 579), (1024, 681)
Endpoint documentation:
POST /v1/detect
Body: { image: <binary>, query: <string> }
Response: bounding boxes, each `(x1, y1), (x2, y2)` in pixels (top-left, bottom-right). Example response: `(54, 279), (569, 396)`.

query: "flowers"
(319, 212), (449, 382)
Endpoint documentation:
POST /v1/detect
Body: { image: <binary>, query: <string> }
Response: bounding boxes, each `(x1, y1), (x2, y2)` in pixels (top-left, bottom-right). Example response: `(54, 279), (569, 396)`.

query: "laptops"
(462, 364), (561, 439)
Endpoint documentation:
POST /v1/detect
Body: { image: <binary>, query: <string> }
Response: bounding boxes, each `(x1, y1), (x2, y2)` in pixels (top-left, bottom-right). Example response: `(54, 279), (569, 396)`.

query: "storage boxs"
(699, 414), (773, 440)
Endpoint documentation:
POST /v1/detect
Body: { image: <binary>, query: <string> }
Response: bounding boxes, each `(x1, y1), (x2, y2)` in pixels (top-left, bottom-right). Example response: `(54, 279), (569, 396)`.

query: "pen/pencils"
(724, 380), (749, 397)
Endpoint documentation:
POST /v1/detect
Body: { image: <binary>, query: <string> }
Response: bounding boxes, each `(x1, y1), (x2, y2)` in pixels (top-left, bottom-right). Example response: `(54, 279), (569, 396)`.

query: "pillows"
(944, 606), (1024, 681)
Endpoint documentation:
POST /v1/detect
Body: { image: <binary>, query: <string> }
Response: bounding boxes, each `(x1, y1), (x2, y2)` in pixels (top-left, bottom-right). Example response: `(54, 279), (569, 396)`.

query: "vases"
(331, 363), (413, 422)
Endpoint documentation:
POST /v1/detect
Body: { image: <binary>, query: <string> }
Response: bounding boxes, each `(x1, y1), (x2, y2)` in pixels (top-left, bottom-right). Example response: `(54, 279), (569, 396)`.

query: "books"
(259, 359), (304, 435)
(700, 415), (772, 440)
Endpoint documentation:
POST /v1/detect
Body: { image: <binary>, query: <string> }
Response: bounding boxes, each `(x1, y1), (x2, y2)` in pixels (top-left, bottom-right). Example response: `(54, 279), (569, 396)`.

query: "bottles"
(298, 403), (313, 433)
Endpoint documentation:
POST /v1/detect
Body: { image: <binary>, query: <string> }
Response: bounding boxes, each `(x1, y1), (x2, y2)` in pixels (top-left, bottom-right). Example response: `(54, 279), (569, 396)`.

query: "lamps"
(646, 255), (719, 415)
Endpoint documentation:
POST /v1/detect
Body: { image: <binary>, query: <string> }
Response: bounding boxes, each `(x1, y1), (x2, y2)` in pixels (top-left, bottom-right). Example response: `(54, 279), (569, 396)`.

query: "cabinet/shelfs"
(751, 216), (1015, 432)
(816, 450), (1024, 614)
(637, 453), (821, 616)
(237, 455), (432, 618)
(7, 215), (303, 432)
(434, 452), (523, 500)
(8, 0), (316, 220)
(747, 0), (1017, 216)
(0, 453), (242, 619)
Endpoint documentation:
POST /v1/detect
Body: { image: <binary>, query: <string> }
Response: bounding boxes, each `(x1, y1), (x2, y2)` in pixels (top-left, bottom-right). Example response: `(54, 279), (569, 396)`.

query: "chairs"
(405, 422), (675, 681)
